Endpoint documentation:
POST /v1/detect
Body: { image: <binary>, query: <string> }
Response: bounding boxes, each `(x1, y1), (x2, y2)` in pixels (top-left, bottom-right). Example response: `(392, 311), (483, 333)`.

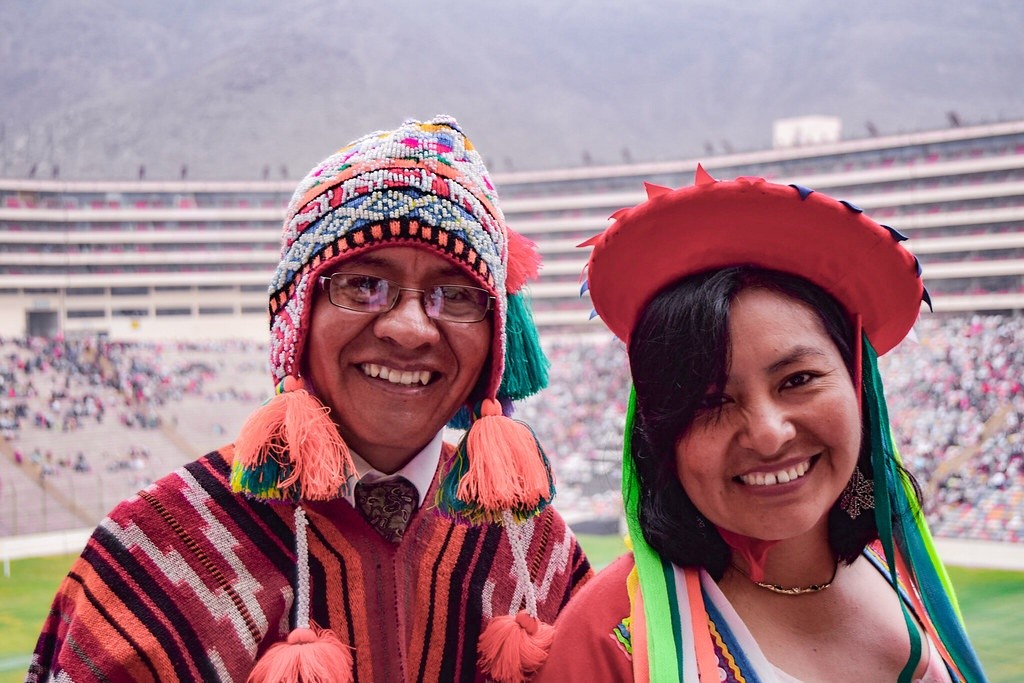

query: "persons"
(2, 314), (1023, 543)
(28, 113), (596, 683)
(533, 164), (986, 682)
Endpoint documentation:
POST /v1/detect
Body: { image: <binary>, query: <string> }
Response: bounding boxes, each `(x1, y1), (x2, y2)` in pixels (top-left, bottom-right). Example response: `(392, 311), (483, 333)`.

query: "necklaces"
(731, 552), (839, 594)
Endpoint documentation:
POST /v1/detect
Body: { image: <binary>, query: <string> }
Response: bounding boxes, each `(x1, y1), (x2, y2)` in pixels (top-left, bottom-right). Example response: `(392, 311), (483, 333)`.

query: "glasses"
(315, 271), (495, 325)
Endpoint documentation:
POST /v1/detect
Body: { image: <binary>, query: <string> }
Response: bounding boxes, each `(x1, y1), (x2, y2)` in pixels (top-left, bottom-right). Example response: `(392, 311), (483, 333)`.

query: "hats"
(265, 114), (514, 404)
(579, 162), (933, 364)
